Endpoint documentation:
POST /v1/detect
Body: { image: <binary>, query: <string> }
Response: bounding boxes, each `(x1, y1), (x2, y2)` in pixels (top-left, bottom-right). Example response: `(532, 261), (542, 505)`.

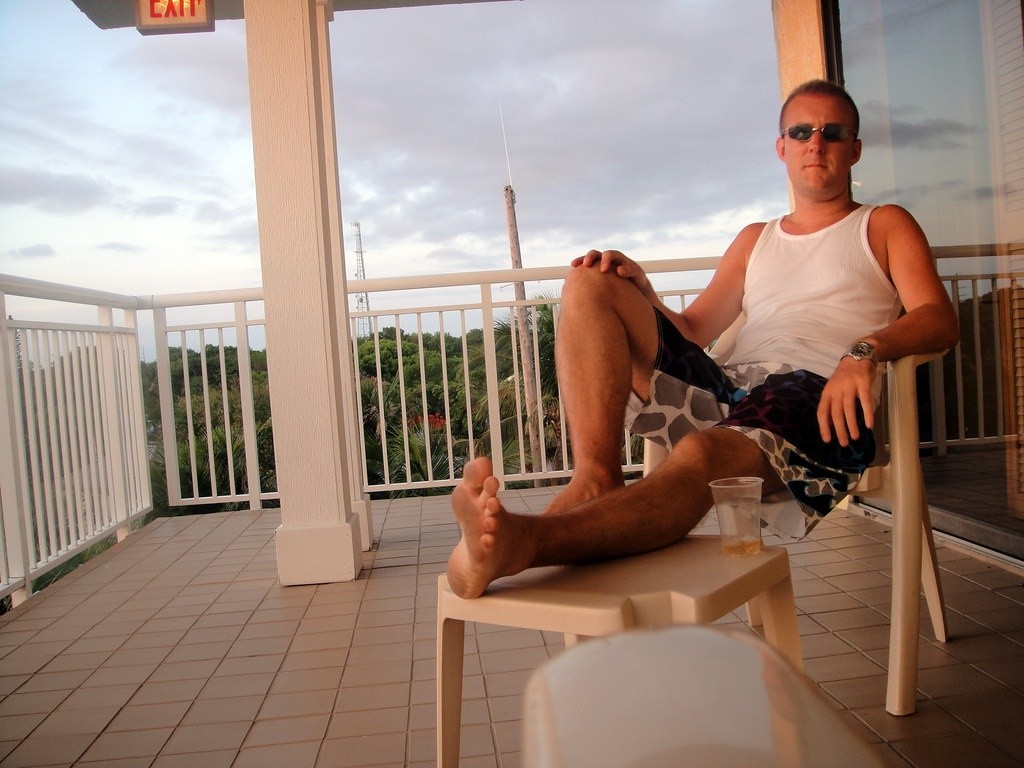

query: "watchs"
(840, 342), (881, 366)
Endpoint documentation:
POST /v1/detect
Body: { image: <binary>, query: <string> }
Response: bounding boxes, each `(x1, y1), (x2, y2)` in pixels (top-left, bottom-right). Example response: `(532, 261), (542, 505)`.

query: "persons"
(448, 80), (958, 600)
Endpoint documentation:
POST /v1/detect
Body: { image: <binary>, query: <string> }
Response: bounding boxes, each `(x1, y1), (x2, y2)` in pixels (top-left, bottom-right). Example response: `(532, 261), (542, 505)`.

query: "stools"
(437, 534), (804, 768)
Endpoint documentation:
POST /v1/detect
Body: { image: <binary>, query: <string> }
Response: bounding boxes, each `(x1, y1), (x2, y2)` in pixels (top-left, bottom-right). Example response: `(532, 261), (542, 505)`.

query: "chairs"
(644, 346), (951, 716)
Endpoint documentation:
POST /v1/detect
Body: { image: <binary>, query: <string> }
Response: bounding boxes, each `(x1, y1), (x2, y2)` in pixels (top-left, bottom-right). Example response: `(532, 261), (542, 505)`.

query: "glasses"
(781, 123), (856, 142)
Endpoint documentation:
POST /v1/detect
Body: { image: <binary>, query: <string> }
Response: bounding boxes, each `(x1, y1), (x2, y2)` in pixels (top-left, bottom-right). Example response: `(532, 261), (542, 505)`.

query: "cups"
(708, 477), (764, 556)
